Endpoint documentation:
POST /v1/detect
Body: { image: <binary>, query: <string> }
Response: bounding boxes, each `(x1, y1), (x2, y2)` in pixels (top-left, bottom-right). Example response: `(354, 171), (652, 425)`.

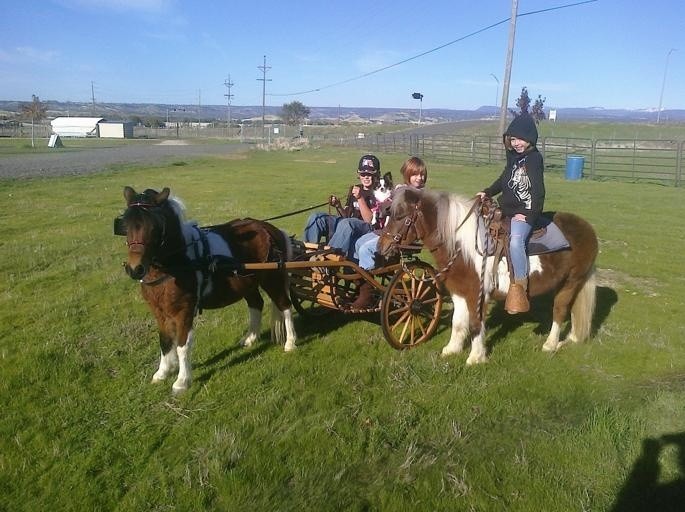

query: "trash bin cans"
(565, 155), (584, 179)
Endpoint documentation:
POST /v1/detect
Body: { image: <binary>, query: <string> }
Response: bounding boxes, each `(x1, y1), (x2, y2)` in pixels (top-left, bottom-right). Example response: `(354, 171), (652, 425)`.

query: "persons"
(300, 149), (388, 275)
(345, 155), (431, 309)
(477, 111), (548, 316)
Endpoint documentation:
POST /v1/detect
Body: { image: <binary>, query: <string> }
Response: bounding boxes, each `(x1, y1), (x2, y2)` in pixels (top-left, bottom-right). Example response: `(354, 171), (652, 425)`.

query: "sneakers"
(514, 277), (530, 292)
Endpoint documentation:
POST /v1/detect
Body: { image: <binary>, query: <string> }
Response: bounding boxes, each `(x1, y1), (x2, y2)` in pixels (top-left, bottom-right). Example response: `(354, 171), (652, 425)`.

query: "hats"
(357, 155), (380, 176)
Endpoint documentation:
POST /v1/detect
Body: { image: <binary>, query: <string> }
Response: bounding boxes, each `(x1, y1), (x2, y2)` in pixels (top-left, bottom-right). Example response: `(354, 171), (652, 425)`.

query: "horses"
(375, 186), (599, 369)
(122, 185), (298, 398)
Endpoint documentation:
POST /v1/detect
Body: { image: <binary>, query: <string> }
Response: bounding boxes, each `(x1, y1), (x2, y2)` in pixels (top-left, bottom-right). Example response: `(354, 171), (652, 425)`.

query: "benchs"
(326, 185), (423, 276)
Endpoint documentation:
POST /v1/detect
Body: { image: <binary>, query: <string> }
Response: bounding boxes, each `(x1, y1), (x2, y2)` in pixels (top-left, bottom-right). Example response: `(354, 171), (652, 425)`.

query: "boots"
(350, 270), (376, 311)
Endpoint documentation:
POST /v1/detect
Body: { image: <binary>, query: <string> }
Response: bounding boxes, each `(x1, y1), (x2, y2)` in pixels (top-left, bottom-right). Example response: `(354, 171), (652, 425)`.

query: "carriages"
(113, 187), (444, 396)
